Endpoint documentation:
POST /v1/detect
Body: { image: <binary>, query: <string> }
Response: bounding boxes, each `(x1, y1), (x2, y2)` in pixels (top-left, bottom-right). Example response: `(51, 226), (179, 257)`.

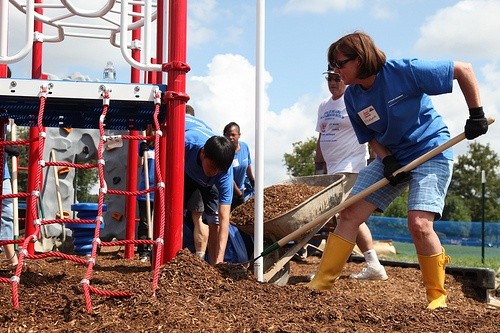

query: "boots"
(415, 247), (452, 310)
(301, 231), (357, 290)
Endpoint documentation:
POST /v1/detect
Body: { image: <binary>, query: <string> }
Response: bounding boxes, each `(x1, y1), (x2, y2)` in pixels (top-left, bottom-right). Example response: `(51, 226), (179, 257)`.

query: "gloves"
(382, 154), (413, 186)
(138, 142), (149, 157)
(464, 106), (488, 140)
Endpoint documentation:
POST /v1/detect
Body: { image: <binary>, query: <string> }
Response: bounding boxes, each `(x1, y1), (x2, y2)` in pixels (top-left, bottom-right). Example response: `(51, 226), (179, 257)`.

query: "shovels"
(214, 119), (494, 282)
(143, 130), (154, 265)
(51, 149), (74, 254)
(34, 194), (54, 253)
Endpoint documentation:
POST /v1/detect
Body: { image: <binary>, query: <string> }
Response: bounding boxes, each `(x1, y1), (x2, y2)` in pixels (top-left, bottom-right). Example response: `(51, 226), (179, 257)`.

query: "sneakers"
(350, 264), (388, 281)
(306, 272), (316, 281)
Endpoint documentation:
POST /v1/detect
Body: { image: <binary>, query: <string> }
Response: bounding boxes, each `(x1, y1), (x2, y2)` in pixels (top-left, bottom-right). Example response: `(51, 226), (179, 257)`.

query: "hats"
(322, 63), (343, 76)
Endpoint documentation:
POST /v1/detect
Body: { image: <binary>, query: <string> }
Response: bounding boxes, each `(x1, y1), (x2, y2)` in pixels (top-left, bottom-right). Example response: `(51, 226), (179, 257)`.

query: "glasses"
(330, 56), (356, 69)
(325, 76), (342, 82)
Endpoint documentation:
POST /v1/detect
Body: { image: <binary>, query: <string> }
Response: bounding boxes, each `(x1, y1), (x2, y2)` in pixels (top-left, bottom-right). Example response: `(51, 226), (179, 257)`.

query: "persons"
(303, 30), (489, 309)
(136, 104), (255, 267)
(0, 123), (19, 267)
(306, 63), (388, 282)
